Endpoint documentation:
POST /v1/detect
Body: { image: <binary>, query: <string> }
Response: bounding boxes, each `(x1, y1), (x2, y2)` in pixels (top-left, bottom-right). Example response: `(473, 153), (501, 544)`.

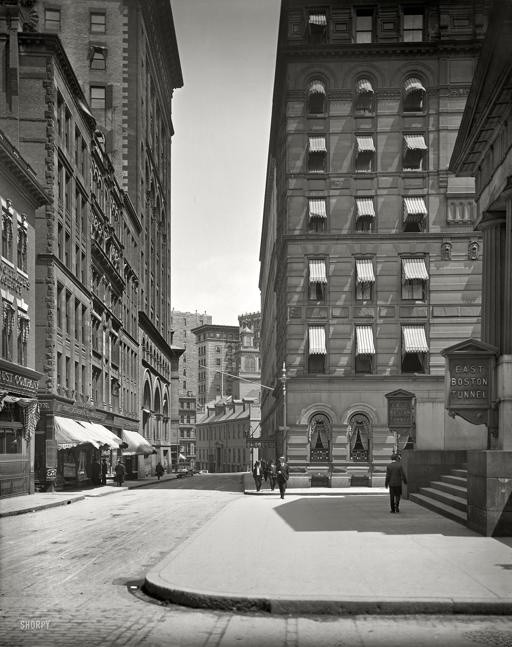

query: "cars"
(176, 468), (193, 479)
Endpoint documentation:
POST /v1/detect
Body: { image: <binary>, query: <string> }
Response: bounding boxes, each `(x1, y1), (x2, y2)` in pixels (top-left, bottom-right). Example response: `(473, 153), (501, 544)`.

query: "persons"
(253, 456), (289, 499)
(156, 463), (164, 480)
(102, 459), (107, 485)
(115, 461), (125, 487)
(385, 454), (407, 513)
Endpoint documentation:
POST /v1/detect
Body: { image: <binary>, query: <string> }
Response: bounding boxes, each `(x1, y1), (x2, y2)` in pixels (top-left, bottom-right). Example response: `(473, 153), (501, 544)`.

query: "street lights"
(279, 362), (291, 475)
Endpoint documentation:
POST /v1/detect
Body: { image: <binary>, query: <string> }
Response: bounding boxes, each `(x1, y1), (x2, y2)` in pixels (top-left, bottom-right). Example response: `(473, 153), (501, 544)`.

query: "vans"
(200, 470), (208, 474)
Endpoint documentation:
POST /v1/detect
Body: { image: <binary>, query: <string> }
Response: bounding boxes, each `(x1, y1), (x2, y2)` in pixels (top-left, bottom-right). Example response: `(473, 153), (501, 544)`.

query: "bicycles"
(116, 475), (123, 486)
